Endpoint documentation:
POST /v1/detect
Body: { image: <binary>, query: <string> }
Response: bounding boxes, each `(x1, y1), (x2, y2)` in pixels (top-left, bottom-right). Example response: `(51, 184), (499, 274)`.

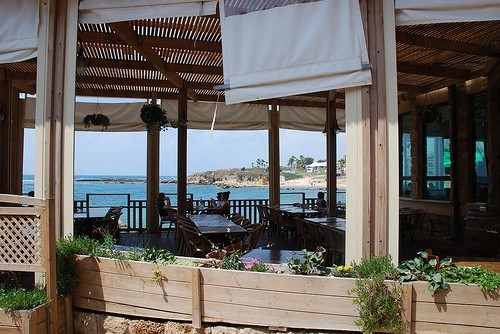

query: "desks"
(71, 207), (110, 219)
(304, 217), (346, 252)
(188, 214), (248, 244)
(267, 205), (319, 222)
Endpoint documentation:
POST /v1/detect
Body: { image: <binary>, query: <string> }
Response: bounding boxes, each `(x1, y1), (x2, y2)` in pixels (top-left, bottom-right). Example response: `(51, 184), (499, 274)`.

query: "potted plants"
(82, 114), (109, 131)
(140, 104), (167, 132)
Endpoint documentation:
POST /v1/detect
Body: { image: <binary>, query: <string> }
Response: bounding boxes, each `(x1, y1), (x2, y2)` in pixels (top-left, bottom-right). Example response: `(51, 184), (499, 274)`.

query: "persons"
(206, 191), (230, 218)
(157, 193), (177, 217)
(317, 192), (326, 206)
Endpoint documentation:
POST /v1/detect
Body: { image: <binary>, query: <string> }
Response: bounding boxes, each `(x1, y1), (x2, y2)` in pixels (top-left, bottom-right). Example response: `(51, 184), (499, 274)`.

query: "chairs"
(98, 203), (471, 268)
